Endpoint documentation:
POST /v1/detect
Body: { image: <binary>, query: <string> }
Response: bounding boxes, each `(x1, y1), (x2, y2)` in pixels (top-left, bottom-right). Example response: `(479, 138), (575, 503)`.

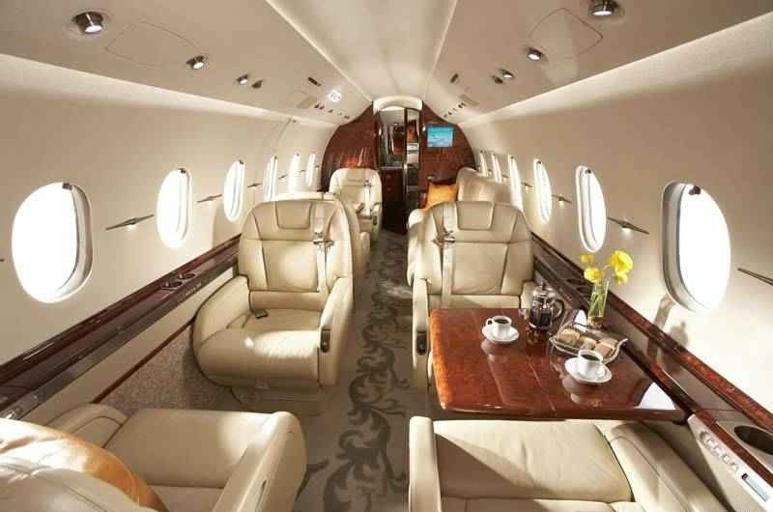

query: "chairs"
(193, 197), (353, 416)
(410, 201), (542, 396)
(404, 166), (512, 286)
(0, 404), (310, 510)
(404, 415), (763, 512)
(328, 165), (385, 245)
(267, 190), (371, 303)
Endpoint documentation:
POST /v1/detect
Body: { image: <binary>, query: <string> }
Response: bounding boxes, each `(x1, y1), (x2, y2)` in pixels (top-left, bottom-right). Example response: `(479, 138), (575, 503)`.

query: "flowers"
(581, 250), (632, 284)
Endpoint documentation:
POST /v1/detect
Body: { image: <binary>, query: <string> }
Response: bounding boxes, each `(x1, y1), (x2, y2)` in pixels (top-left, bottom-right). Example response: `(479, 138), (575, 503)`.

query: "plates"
(564, 357), (612, 383)
(481, 325), (519, 344)
(549, 321), (628, 364)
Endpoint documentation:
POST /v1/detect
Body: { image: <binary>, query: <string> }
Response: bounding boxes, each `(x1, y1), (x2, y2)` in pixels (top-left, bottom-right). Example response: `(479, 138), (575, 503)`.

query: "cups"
(486, 315), (512, 339)
(577, 349), (603, 380)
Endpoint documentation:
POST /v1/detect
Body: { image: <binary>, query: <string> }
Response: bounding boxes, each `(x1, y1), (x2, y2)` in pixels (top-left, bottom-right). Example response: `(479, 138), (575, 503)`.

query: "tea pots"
(529, 281), (565, 330)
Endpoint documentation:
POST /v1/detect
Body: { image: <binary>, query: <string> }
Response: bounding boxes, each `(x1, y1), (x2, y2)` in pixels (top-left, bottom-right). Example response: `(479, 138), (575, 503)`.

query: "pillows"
(422, 184), (460, 210)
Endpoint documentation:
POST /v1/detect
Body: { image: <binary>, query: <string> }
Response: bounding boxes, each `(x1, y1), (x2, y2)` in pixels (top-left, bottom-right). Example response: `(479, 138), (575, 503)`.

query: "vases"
(586, 278), (609, 328)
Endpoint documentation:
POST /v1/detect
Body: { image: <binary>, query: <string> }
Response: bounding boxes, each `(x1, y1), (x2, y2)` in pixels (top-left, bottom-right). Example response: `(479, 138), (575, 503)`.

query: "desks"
(353, 201), (364, 214)
